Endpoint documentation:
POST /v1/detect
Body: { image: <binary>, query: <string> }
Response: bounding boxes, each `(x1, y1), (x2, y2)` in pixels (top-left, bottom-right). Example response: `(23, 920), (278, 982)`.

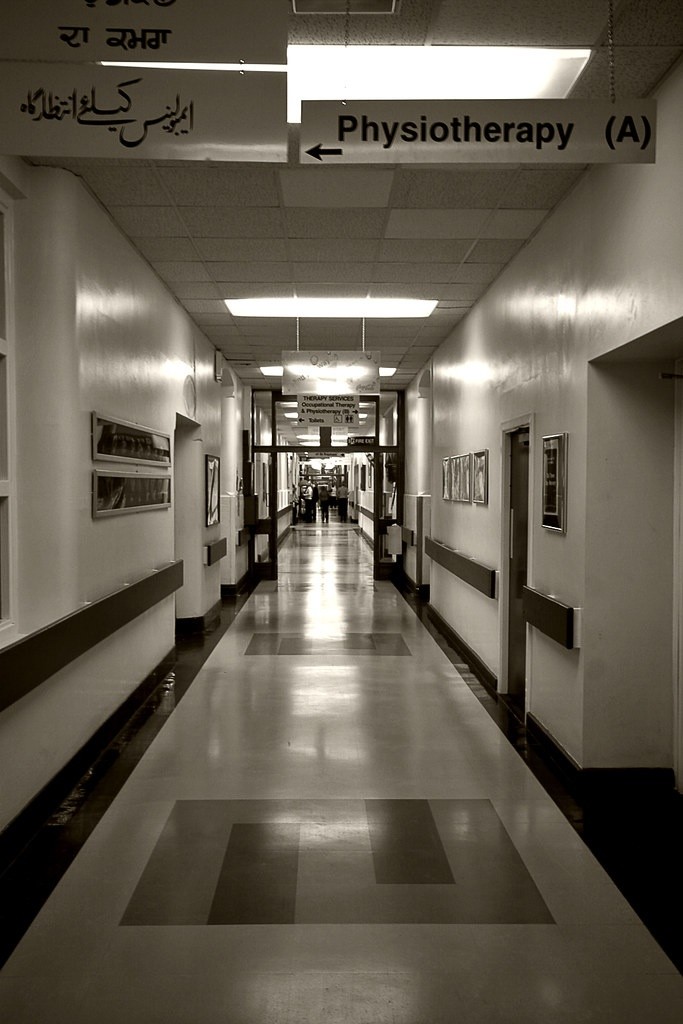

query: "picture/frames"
(539, 432), (567, 533)
(442, 449), (490, 503)
(205, 453), (221, 527)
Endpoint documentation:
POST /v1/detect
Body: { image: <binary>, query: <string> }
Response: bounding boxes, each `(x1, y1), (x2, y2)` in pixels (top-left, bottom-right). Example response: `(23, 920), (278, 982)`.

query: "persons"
(291, 475), (352, 524)
